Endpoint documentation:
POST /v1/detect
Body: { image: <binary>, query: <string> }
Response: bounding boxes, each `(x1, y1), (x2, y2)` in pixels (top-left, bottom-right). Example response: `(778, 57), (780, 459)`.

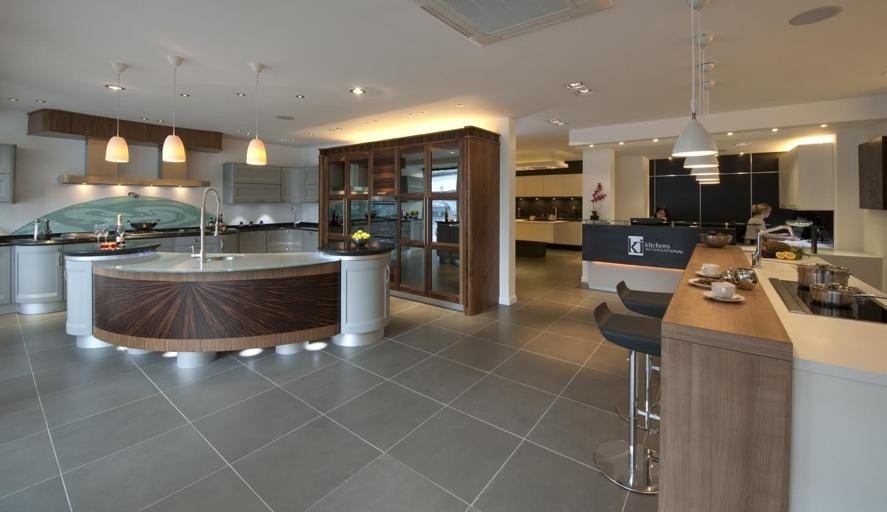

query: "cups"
(733, 268), (758, 289)
(701, 263), (720, 275)
(711, 282), (736, 299)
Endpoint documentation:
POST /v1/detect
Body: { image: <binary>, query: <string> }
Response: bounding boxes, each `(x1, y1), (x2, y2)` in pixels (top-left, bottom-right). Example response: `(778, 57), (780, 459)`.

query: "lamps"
(160, 56), (188, 164)
(244, 62), (269, 168)
(669, 0), (722, 187)
(103, 63), (133, 164)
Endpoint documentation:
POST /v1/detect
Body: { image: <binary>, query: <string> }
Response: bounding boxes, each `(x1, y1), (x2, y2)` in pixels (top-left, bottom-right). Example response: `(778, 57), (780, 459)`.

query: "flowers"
(591, 182), (607, 209)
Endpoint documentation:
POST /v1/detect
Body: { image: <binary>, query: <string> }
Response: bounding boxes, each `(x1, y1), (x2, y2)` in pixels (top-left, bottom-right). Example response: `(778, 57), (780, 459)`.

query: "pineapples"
(761, 241), (811, 259)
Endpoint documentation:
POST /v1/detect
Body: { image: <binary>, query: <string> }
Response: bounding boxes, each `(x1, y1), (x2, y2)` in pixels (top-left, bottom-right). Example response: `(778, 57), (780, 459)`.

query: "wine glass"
(103, 224), (109, 242)
(94, 224), (101, 244)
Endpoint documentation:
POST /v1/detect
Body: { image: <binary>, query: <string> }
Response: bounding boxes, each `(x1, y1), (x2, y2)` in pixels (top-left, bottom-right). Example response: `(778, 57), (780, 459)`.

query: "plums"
(100, 242), (116, 248)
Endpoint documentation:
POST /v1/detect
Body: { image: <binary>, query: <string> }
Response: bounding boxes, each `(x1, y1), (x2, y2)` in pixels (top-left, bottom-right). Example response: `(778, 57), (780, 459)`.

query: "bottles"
(115, 215), (126, 247)
(33, 220), (40, 241)
(43, 219), (50, 233)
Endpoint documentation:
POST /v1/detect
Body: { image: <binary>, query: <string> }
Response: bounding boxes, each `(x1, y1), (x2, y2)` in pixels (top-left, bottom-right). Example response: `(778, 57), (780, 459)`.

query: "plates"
(696, 270), (722, 278)
(703, 290), (745, 302)
(688, 278), (735, 288)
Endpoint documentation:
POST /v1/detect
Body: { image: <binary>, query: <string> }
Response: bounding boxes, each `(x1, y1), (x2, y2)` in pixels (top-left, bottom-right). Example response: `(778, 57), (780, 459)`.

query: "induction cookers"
(769, 278), (886, 324)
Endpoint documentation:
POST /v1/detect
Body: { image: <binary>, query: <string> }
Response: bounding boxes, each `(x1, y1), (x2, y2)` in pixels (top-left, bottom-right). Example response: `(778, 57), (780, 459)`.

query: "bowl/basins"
(705, 234), (730, 248)
(699, 232), (733, 245)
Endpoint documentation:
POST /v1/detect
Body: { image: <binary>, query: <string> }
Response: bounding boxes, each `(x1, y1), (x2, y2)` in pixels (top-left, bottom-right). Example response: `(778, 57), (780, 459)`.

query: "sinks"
(198, 255), (238, 263)
(760, 257), (798, 283)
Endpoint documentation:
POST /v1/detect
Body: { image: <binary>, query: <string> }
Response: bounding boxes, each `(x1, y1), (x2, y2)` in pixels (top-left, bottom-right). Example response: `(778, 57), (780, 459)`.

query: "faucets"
(750, 223), (795, 265)
(198, 186), (220, 263)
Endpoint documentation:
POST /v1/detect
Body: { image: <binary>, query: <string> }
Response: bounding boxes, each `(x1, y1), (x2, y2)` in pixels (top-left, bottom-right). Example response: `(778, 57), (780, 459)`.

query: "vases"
(589, 210), (599, 220)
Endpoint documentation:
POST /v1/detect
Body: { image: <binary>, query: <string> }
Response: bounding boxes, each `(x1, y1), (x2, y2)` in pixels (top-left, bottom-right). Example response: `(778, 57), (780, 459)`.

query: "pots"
(810, 282), (887, 306)
(126, 219), (160, 231)
(797, 263), (852, 289)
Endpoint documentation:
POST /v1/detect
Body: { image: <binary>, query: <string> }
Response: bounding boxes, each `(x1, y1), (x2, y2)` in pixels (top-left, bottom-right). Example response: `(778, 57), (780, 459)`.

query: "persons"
(651, 206), (672, 224)
(743, 203), (773, 245)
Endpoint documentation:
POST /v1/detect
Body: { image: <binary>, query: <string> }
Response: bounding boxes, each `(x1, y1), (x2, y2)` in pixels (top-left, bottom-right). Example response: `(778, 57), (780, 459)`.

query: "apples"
(352, 230), (370, 240)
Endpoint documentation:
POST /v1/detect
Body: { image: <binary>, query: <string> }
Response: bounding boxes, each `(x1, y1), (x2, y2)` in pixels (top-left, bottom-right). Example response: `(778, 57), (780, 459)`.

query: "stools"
(592, 300), (663, 498)
(613, 280), (675, 434)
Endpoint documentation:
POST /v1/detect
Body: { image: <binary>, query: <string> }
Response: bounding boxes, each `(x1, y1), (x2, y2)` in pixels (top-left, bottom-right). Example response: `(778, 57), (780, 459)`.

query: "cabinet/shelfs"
(173, 234), (239, 254)
(316, 125), (503, 318)
(14, 245), (63, 304)
(130, 237), (174, 253)
(777, 143), (836, 212)
(266, 227), (319, 253)
(515, 218), (571, 260)
(61, 243), (99, 303)
(0, 143), (18, 205)
(547, 221), (584, 252)
(220, 161), (319, 206)
(238, 231), (267, 253)
(0, 243), (13, 307)
